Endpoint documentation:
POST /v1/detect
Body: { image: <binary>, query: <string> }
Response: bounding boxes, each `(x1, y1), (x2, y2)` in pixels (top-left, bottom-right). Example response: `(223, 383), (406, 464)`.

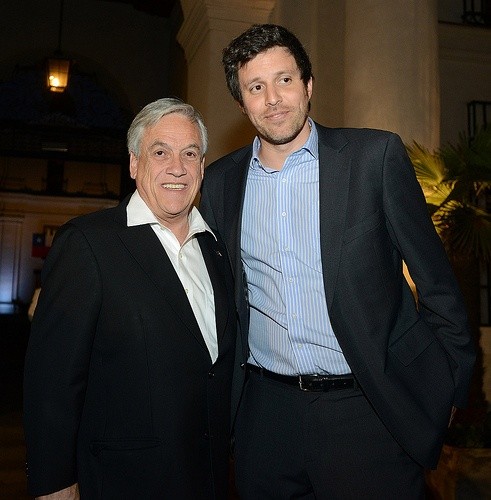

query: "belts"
(243, 363), (359, 394)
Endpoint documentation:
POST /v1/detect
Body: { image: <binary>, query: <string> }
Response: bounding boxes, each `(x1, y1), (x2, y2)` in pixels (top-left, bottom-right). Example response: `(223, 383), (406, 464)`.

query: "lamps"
(46, 3), (75, 94)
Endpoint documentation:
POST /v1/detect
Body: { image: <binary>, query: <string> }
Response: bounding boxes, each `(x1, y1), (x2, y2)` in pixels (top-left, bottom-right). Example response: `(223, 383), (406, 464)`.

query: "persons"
(23, 97), (236, 500)
(198, 23), (477, 500)
